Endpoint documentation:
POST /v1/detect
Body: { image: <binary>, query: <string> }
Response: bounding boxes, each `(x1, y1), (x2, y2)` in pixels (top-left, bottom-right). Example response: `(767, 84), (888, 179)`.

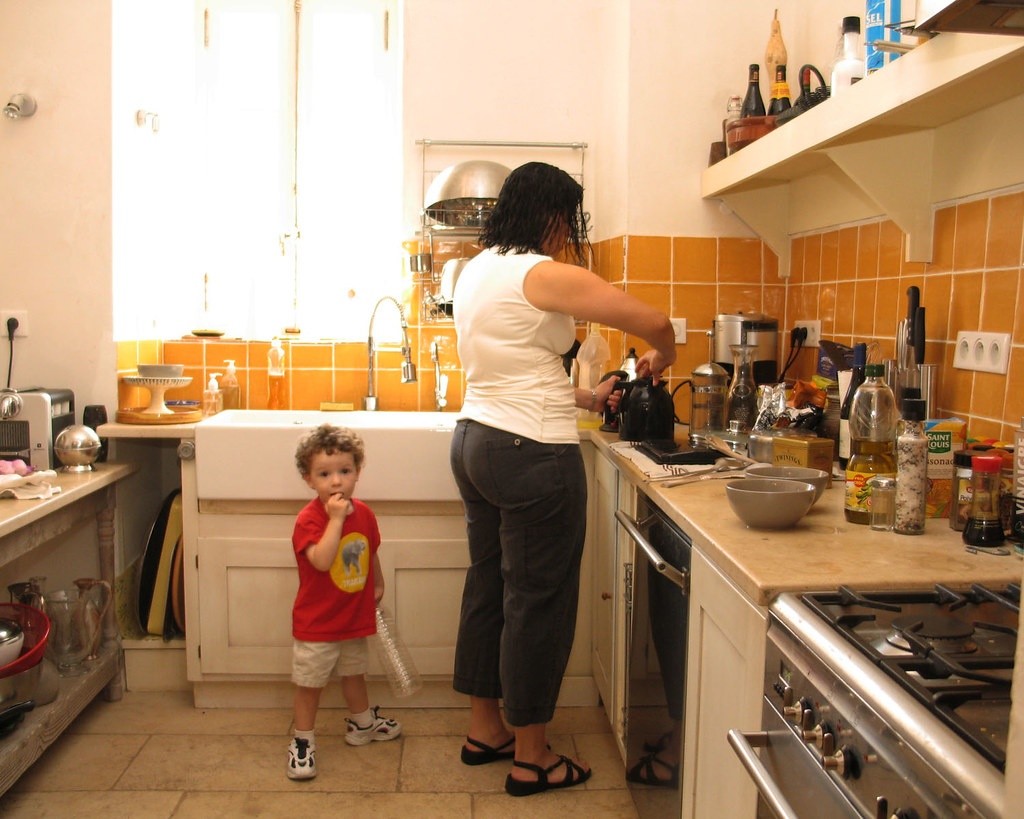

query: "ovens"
(727, 624), (862, 819)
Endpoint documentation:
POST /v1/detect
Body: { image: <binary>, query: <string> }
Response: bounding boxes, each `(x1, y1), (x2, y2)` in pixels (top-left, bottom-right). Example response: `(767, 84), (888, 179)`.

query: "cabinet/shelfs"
(680, 541), (769, 819)
(98, 419), (192, 693)
(589, 445), (623, 742)
(198, 536), (473, 675)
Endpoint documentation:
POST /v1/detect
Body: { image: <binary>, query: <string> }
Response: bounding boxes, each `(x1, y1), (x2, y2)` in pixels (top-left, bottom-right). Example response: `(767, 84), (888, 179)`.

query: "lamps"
(4, 93), (38, 121)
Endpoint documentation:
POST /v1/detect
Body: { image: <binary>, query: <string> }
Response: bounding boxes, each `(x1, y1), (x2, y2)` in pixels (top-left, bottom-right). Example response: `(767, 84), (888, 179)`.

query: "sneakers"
(285, 736), (317, 780)
(344, 705), (400, 746)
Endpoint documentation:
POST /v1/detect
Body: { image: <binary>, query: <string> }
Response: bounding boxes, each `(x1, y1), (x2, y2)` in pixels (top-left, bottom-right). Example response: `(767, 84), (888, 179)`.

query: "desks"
(0, 461), (139, 797)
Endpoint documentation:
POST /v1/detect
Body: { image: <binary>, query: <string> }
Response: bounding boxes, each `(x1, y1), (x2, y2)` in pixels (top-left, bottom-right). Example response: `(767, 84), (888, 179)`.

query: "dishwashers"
(615, 486), (692, 819)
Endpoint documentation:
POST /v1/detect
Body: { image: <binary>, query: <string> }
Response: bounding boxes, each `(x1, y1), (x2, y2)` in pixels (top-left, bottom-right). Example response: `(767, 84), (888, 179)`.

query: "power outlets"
(2, 310), (28, 338)
(793, 321), (820, 346)
(669, 318), (687, 343)
(952, 330), (1013, 375)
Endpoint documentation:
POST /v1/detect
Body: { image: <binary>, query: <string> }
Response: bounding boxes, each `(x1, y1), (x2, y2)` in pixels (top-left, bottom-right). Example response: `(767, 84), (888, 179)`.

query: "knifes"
(904, 285), (925, 371)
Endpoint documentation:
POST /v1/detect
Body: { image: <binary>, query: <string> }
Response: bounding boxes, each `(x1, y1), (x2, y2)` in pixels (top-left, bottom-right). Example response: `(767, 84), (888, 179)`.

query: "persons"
(286, 426), (401, 780)
(447, 162), (677, 798)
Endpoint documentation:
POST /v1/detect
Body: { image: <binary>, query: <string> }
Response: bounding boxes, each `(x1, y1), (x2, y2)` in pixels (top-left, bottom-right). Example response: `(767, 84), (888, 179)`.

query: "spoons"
(705, 433), (759, 464)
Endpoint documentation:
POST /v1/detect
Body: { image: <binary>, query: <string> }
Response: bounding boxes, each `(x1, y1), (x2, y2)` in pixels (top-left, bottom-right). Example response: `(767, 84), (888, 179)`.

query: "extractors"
(915, 0), (1024, 36)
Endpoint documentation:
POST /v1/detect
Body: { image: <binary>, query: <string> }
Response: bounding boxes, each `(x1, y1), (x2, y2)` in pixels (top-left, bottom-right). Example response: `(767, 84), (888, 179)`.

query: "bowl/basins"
(436, 257), (472, 315)
(744, 467), (829, 506)
(423, 160), (513, 226)
(138, 364), (184, 378)
(725, 479), (816, 532)
(0, 602), (52, 678)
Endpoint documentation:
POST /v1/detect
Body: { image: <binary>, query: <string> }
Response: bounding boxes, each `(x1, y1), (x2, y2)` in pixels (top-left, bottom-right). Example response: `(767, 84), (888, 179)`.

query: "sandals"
(460, 733), (551, 765)
(505, 754), (591, 797)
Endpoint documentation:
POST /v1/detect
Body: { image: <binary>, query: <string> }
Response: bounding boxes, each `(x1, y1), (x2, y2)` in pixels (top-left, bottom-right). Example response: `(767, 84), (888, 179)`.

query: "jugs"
(7, 576), (112, 679)
(672, 360), (731, 448)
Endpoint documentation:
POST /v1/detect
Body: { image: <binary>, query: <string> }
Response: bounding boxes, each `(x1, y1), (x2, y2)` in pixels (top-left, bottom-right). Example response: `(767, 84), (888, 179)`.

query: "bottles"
(767, 65), (792, 116)
(844, 363), (897, 525)
(725, 96), (743, 157)
(369, 608), (423, 698)
(951, 438), (1015, 548)
(1011, 417), (1024, 549)
(266, 339), (286, 410)
(740, 64), (766, 119)
(830, 16), (866, 97)
(892, 386), (928, 535)
(871, 478), (897, 532)
(724, 344), (759, 431)
(793, 67), (810, 106)
(576, 323), (610, 430)
(839, 343), (866, 471)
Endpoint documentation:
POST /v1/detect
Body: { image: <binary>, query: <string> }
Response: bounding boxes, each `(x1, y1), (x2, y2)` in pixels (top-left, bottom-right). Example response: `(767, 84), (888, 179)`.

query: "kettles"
(604, 377), (675, 441)
(599, 347), (640, 433)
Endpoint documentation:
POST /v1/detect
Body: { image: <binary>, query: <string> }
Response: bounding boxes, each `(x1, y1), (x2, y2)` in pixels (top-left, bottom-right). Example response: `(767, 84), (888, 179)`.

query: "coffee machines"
(711, 311), (779, 387)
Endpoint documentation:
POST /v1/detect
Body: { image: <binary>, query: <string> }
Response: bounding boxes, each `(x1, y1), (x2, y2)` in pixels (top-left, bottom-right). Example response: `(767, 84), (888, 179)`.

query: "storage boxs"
(773, 434), (833, 488)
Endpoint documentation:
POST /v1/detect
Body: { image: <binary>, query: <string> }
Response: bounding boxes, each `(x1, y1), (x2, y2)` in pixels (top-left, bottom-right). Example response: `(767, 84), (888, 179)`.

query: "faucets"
(431, 341), (449, 411)
(362, 296), (418, 410)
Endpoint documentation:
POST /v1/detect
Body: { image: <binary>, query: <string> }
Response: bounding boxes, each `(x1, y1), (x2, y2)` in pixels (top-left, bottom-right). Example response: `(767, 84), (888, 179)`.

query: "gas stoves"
(768, 584), (1024, 819)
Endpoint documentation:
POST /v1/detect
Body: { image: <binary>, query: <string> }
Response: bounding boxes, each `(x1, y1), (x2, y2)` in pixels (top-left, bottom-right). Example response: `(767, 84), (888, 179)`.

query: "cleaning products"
(267, 339), (288, 411)
(202, 359), (241, 414)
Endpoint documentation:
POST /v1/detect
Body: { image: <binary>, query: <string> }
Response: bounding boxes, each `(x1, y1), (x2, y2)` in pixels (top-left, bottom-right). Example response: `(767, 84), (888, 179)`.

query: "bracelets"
(586, 390), (597, 413)
